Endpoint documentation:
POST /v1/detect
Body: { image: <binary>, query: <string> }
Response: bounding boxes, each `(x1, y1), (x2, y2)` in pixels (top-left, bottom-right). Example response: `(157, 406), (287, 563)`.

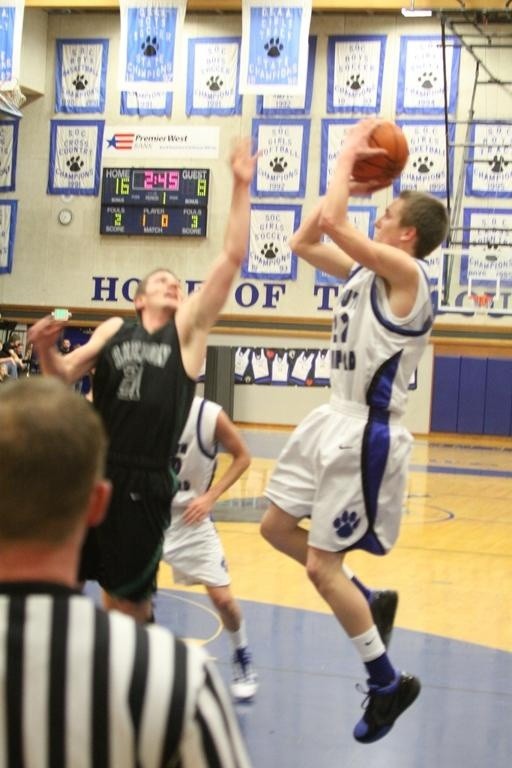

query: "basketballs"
(343, 119), (409, 191)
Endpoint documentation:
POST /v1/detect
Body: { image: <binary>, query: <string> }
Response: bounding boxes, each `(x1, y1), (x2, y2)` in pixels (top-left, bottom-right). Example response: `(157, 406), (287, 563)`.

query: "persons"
(1, 372), (251, 768)
(28, 140), (256, 625)
(0, 333), (96, 396)
(260, 121), (450, 744)
(160, 395), (257, 701)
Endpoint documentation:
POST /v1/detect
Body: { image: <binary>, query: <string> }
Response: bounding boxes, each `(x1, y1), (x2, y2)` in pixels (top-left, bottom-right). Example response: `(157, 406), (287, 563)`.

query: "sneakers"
(370, 587), (397, 653)
(348, 669), (420, 746)
(229, 645), (261, 703)
